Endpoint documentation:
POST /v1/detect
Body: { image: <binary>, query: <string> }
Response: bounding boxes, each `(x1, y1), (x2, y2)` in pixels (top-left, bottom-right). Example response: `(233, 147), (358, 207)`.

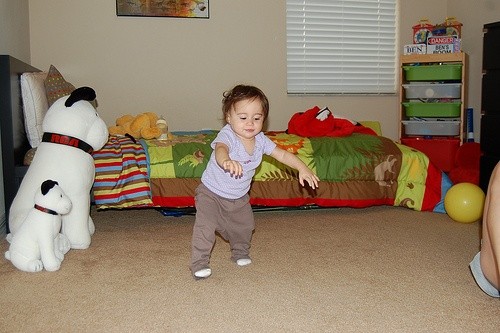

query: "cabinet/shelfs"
(478, 21), (500, 193)
(397, 52), (469, 147)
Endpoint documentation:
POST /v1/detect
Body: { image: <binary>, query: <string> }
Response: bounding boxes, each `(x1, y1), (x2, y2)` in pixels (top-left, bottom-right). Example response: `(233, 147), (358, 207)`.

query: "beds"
(0, 51), (480, 233)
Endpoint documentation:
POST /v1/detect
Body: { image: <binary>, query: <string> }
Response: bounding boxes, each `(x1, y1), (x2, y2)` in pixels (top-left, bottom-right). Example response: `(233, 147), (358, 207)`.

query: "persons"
(189, 83), (321, 278)
(468, 159), (500, 298)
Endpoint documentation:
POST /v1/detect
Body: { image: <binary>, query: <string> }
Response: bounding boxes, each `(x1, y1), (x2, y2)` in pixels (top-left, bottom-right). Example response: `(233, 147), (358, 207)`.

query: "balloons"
(443, 182), (485, 223)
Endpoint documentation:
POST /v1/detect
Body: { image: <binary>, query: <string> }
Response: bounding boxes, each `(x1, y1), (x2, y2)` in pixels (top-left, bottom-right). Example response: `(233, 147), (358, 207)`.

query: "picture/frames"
(115, 0), (210, 19)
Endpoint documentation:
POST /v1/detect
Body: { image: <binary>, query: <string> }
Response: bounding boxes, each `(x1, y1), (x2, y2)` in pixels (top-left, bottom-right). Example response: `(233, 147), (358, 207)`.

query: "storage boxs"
(401, 83), (462, 99)
(400, 121), (461, 137)
(404, 44), (426, 52)
(401, 101), (462, 117)
(427, 35), (463, 53)
(402, 64), (462, 81)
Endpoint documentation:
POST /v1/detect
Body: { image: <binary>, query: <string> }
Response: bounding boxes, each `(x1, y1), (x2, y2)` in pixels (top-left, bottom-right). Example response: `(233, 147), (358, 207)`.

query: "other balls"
(443, 182), (486, 223)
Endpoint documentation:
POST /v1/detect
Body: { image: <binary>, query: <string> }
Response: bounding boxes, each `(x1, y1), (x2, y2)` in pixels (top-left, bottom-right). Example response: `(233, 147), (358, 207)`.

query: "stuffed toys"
(6, 85), (108, 249)
(109, 112), (173, 142)
(5, 180), (74, 271)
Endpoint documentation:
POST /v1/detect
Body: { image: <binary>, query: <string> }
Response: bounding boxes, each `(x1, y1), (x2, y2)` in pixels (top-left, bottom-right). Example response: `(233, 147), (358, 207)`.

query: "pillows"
(44, 63), (98, 112)
